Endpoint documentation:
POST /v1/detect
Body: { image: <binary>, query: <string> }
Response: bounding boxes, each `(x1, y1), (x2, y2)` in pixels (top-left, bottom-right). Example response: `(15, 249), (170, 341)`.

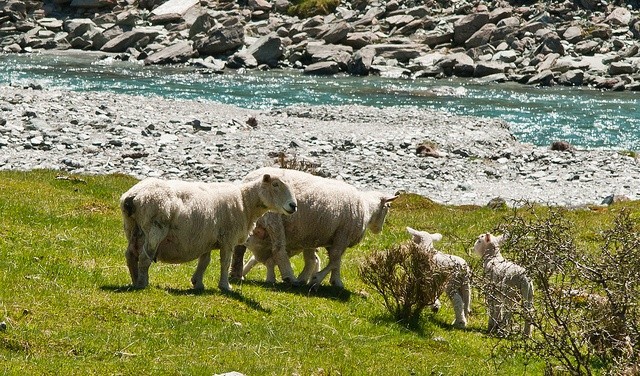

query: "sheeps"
(120, 173), (297, 291)
(242, 223), (320, 292)
(227, 166), (398, 293)
(405, 226), (470, 329)
(474, 232), (534, 338)
